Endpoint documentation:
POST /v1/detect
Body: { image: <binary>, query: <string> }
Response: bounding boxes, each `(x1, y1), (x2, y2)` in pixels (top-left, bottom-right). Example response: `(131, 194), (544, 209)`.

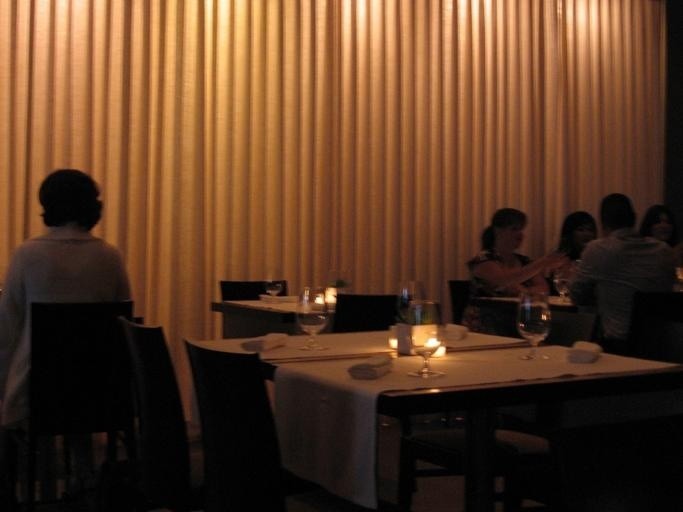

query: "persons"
(542, 209), (599, 298)
(637, 203), (683, 269)
(0, 169), (131, 431)
(460, 206), (571, 337)
(565, 191), (677, 352)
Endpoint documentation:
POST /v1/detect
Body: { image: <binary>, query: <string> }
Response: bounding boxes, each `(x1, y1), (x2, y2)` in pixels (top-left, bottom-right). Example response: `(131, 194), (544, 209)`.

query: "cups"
(398, 279), (428, 325)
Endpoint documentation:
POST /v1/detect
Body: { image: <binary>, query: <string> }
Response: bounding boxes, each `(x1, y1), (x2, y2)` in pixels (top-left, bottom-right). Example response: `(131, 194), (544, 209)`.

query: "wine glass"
(296, 286), (328, 353)
(265, 271), (284, 305)
(407, 298), (446, 379)
(553, 267), (570, 303)
(516, 291), (554, 361)
